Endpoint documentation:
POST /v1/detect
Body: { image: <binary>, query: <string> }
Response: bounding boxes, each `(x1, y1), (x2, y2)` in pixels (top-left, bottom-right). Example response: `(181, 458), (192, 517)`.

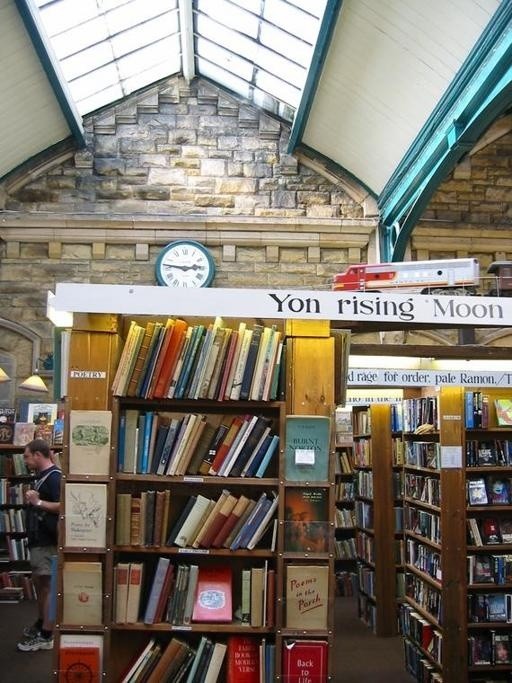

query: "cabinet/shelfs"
(337, 312), (512, 683)
(0, 311), (338, 683)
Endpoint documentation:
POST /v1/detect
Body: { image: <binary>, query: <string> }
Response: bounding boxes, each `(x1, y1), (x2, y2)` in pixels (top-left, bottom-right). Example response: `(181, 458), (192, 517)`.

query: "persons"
(16, 439), (62, 652)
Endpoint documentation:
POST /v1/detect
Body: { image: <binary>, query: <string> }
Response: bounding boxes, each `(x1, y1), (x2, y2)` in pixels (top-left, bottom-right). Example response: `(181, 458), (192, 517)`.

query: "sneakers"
(16, 632), (54, 651)
(23, 623), (43, 637)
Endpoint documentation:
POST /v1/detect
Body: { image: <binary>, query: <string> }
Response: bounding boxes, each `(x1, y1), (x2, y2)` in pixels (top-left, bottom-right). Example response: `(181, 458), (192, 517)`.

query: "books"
(122, 635), (276, 683)
(281, 639), (329, 683)
(115, 485), (279, 552)
(69, 409), (112, 475)
(334, 407), (377, 636)
(62, 562), (103, 625)
(65, 483), (107, 548)
(464, 391), (512, 680)
(110, 316), (286, 402)
(58, 635), (104, 683)
(286, 566), (329, 630)
(113, 555), (276, 629)
(284, 486), (330, 553)
(285, 415), (330, 481)
(53, 326), (72, 402)
(117, 409), (279, 478)
(390, 395), (442, 683)
(1, 408), (64, 604)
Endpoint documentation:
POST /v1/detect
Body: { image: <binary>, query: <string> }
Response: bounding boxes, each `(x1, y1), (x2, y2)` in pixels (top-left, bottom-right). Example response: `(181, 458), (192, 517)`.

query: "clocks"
(155, 238), (216, 287)
